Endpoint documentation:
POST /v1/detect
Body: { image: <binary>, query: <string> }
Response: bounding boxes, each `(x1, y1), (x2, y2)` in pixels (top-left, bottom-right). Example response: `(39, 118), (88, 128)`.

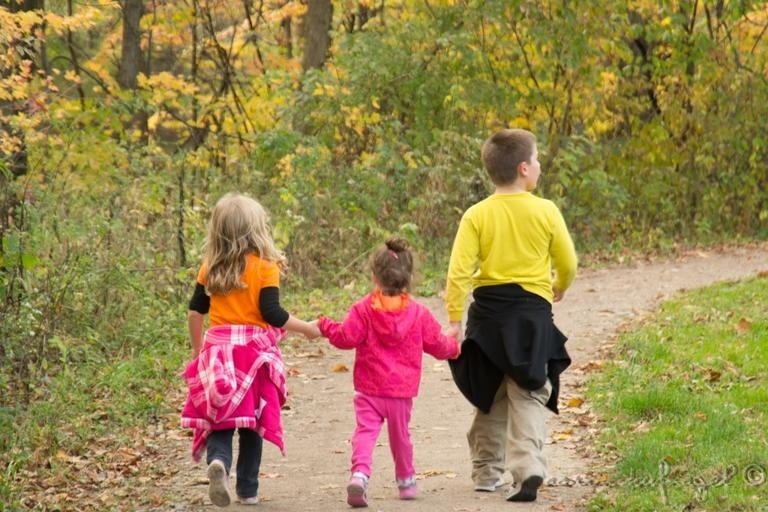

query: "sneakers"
(507, 474), (542, 501)
(235, 494), (257, 505)
(206, 461), (231, 507)
(347, 473), (368, 506)
(475, 480), (504, 491)
(399, 484), (416, 498)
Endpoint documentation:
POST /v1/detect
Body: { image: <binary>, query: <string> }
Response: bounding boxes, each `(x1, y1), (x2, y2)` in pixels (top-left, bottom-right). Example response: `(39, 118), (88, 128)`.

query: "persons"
(445, 128), (577, 503)
(185, 191), (323, 507)
(310, 239), (461, 506)
